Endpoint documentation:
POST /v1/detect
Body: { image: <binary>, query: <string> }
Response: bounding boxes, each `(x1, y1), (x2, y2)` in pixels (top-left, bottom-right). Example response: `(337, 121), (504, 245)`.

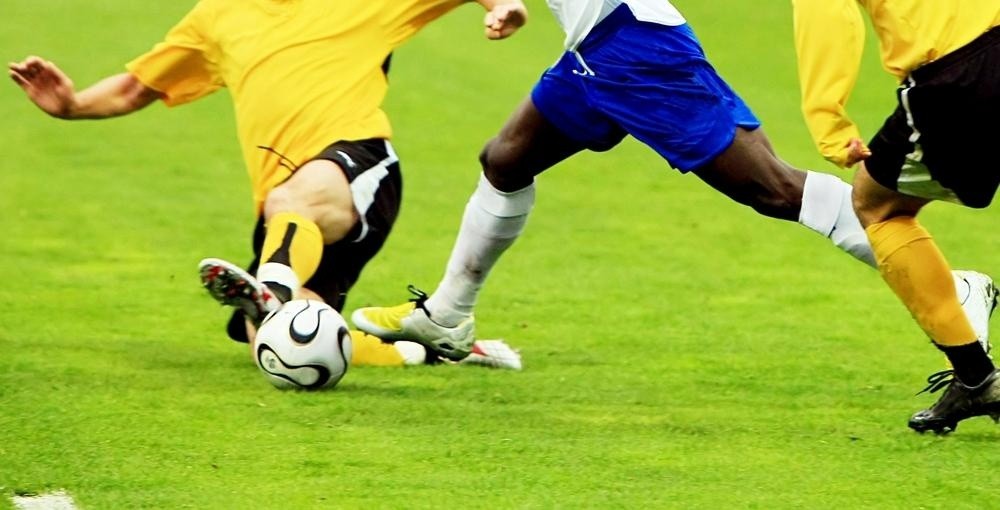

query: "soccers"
(253, 298), (352, 391)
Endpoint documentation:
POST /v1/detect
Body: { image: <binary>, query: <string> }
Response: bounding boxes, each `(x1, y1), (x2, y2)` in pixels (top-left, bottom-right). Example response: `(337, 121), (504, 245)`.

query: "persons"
(790, 0), (1000, 435)
(347, 1), (999, 388)
(4, 0), (531, 369)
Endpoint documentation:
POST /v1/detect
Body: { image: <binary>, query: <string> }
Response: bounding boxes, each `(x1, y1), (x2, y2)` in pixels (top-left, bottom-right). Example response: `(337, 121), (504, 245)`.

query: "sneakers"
(952, 270), (999, 361)
(350, 299), (475, 361)
(197, 257), (291, 330)
(908, 368), (999, 434)
(460, 340), (522, 370)
